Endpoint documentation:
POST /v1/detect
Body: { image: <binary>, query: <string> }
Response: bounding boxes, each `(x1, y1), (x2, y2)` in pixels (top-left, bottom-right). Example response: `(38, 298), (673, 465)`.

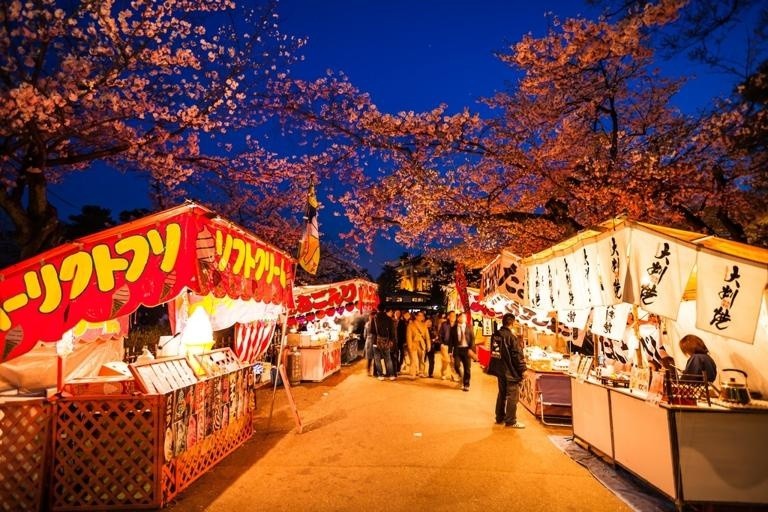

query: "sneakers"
(464, 386), (470, 391)
(441, 376), (445, 380)
(507, 421), (526, 427)
(378, 376), (397, 380)
(411, 373), (433, 381)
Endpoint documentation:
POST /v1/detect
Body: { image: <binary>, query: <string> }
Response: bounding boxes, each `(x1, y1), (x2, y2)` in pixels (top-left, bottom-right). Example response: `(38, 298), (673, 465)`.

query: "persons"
(484, 311), (529, 429)
(335, 307), (477, 391)
(678, 333), (717, 387)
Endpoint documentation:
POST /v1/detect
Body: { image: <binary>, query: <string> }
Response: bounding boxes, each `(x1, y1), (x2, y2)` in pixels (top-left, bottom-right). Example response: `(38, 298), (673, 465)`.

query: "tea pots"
(709, 367), (751, 403)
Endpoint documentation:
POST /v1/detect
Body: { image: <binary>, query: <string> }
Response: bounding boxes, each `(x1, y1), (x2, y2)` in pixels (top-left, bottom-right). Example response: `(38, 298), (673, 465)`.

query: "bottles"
(671, 384), (697, 405)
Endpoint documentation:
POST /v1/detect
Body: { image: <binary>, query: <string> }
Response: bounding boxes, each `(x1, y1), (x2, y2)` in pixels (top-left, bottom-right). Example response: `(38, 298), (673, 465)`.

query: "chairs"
(535, 374), (573, 427)
